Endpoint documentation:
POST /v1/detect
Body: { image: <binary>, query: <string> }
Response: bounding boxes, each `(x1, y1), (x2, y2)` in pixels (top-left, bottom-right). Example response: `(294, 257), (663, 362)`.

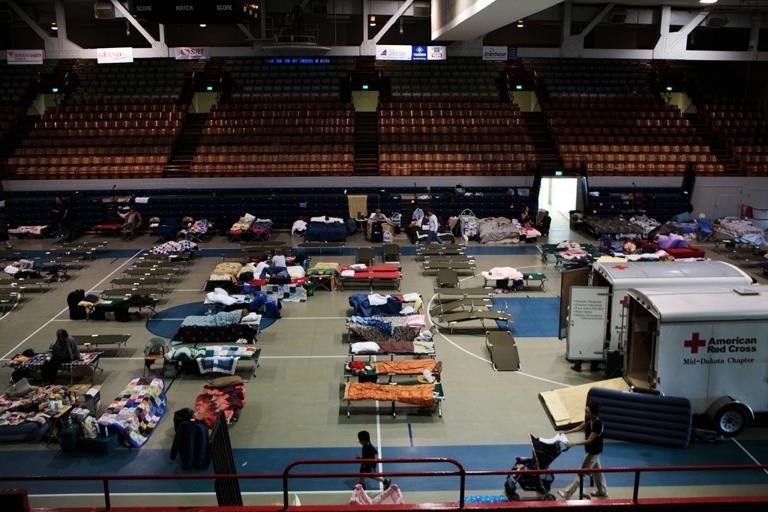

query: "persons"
(520, 203), (534, 230)
(369, 209), (387, 221)
(3, 265), (42, 279)
(514, 463), (532, 471)
(425, 208), (444, 244)
(116, 206), (143, 228)
(47, 196), (67, 237)
(556, 401), (607, 500)
(0, 180), (13, 249)
(66, 289), (87, 320)
(10, 259), (49, 270)
(353, 429), (392, 491)
(39, 329), (82, 384)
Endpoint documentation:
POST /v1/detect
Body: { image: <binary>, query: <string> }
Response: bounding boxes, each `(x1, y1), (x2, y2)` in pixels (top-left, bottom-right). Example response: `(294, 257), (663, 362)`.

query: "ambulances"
(559, 259), (753, 362)
(540, 285), (768, 438)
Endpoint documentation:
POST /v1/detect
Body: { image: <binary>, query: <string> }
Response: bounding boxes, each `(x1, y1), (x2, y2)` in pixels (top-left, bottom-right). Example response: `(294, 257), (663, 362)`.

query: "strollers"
(503, 433), (569, 501)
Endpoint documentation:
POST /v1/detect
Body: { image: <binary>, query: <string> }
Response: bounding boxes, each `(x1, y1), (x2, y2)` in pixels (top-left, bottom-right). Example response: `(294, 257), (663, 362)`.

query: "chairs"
(3, 65), (767, 178)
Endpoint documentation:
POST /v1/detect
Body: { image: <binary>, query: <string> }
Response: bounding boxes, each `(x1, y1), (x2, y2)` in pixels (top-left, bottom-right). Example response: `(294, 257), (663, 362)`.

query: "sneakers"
(557, 489), (609, 500)
(353, 478), (391, 492)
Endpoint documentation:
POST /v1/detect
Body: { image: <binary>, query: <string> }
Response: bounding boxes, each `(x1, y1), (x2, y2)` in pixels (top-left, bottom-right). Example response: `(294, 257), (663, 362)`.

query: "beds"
(343, 357), (442, 386)
(1, 211), (309, 446)
(442, 302), (513, 334)
(338, 379), (446, 420)
(347, 338), (437, 359)
(344, 313), (425, 342)
(313, 205), (552, 313)
(483, 328), (522, 373)
(533, 208), (768, 282)
